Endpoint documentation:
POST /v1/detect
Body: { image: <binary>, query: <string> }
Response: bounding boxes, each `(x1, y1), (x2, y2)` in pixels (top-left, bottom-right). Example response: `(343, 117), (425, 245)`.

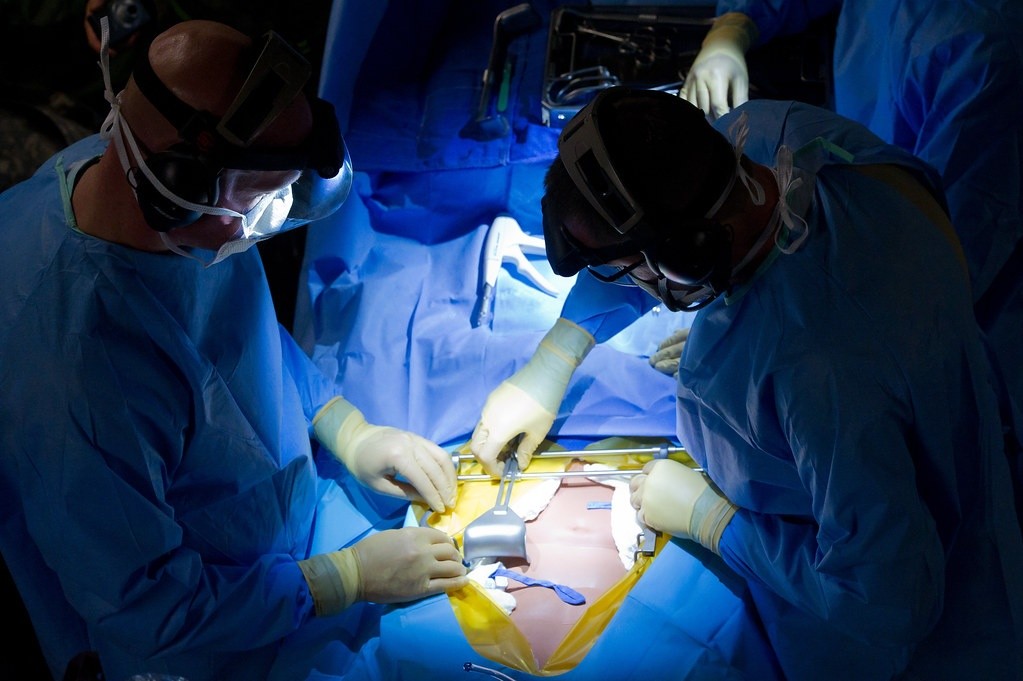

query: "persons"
(0, 19), (470, 681)
(470, 88), (1023, 681)
(679, 0), (1023, 443)
(478, 457), (628, 668)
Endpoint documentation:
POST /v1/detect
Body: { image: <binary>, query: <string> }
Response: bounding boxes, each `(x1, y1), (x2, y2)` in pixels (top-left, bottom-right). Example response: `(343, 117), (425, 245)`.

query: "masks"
(622, 266), (665, 305)
(203, 183), (294, 270)
